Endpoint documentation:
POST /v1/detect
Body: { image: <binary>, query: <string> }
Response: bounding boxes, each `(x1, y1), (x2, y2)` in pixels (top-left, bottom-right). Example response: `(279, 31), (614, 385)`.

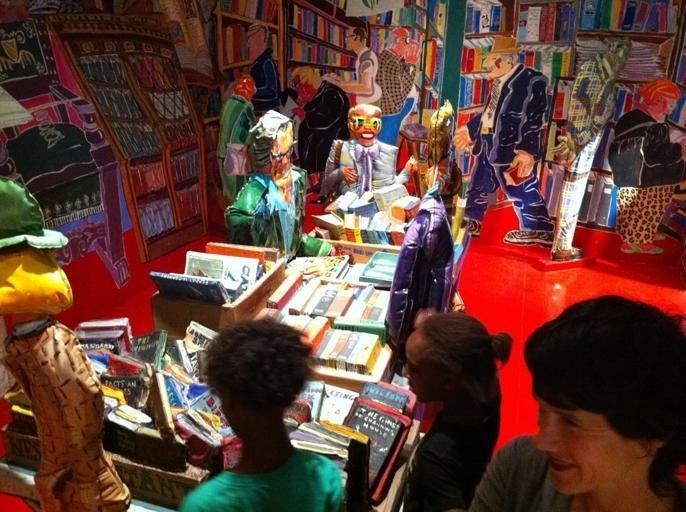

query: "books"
(74, 185), (425, 503)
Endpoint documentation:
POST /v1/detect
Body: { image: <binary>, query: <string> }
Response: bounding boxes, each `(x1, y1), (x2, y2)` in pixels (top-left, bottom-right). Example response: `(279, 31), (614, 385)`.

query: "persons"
(464, 293), (686, 512)
(396, 308), (518, 512)
(171, 315), (353, 512)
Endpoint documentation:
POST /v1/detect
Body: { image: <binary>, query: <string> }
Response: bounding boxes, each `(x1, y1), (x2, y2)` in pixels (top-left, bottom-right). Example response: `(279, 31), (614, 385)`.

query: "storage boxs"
(151, 257), (288, 340)
